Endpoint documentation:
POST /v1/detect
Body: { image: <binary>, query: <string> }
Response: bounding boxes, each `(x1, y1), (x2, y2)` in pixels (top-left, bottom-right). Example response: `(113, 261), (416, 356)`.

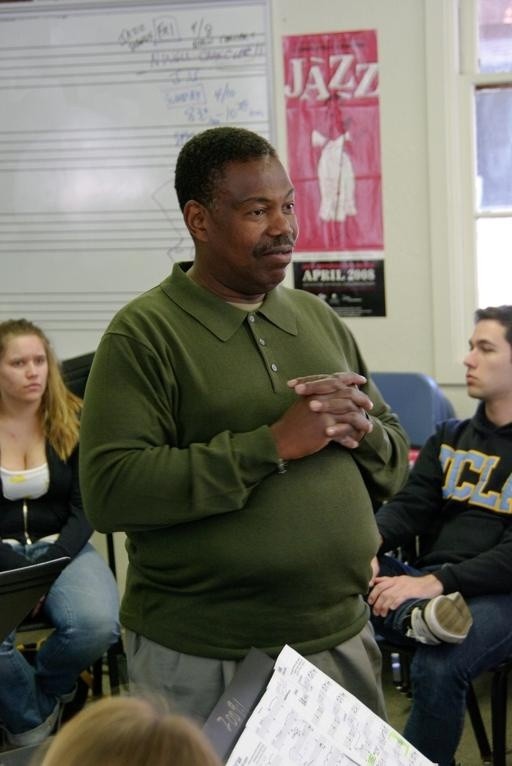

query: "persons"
(0, 318), (121, 755)
(368, 304), (511, 766)
(79, 128), (411, 742)
(26, 692), (227, 766)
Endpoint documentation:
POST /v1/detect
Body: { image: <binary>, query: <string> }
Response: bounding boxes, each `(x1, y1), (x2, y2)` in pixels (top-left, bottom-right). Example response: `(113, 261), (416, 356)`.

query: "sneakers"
(410, 591), (473, 646)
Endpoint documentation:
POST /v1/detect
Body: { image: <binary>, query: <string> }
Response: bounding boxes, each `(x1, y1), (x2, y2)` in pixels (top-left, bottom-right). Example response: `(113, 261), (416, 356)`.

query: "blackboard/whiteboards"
(2, 0), (282, 365)
